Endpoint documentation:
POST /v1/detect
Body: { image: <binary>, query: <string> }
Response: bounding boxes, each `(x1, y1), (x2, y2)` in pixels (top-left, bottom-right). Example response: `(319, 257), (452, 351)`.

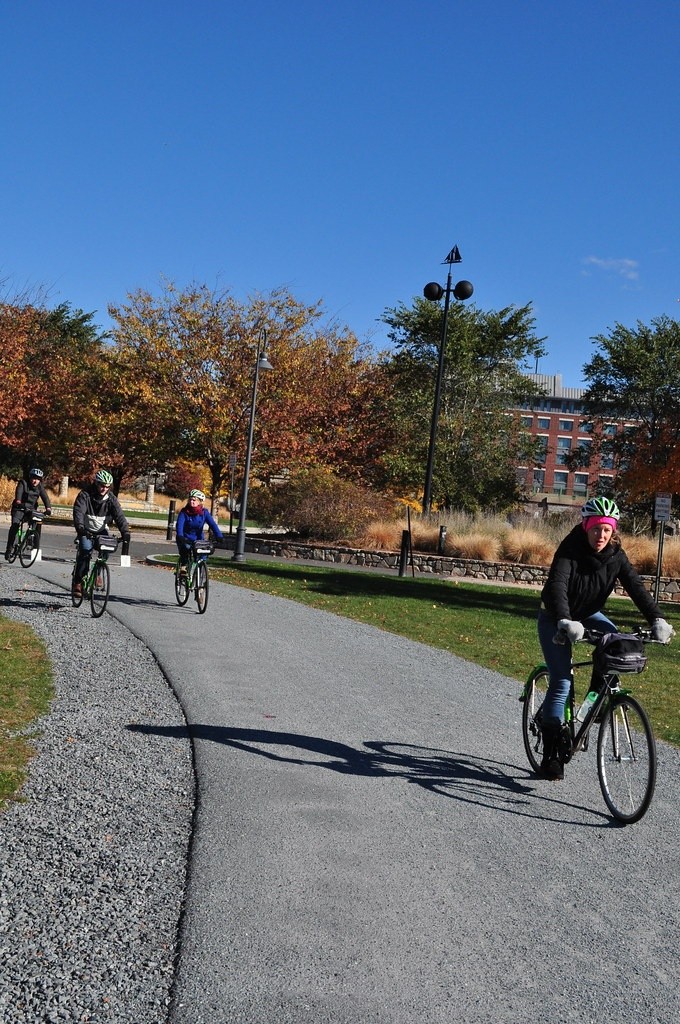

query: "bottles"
(575, 691), (599, 723)
(18, 526), (21, 536)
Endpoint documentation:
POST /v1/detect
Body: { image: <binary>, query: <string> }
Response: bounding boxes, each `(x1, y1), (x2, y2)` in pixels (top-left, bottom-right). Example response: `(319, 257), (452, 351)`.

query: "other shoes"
(74, 584), (82, 597)
(199, 593), (203, 604)
(180, 569), (187, 575)
(5, 550), (10, 560)
(95, 576), (102, 587)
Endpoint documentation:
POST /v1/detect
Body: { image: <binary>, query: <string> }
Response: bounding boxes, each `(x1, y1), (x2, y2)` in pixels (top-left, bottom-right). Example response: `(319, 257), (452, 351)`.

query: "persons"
(176, 489), (225, 604)
(5, 469), (51, 560)
(538, 497), (677, 779)
(73, 470), (131, 597)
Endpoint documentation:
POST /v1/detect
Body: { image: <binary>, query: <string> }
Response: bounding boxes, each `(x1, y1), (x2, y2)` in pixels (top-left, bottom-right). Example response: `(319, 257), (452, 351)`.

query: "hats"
(585, 516), (617, 532)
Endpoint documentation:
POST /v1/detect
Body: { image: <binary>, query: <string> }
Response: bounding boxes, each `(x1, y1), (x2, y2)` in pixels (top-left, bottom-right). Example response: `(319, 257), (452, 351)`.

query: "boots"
(540, 717), (566, 778)
(584, 665), (606, 722)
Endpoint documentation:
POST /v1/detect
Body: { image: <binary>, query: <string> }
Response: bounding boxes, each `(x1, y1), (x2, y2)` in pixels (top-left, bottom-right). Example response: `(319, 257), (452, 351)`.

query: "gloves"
(557, 619), (585, 643)
(651, 617), (671, 641)
(119, 534), (130, 542)
(16, 503), (25, 508)
(216, 537), (225, 543)
(177, 537), (187, 545)
(77, 529), (88, 537)
(42, 510), (51, 516)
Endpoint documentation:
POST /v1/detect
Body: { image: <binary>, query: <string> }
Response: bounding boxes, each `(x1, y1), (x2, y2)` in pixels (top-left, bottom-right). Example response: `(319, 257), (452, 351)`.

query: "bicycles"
(173, 536), (220, 614)
(512, 626), (670, 825)
(5, 503), (52, 568)
(70, 527), (128, 620)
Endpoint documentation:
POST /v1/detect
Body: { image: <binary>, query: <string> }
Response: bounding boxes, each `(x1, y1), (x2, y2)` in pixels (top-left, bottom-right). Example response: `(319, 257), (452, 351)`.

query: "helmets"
(189, 489), (206, 501)
(581, 496), (620, 520)
(94, 470), (114, 486)
(30, 469), (44, 478)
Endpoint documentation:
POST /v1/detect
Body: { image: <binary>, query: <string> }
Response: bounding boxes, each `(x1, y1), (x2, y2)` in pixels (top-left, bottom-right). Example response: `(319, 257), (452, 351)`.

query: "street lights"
(415, 246), (474, 514)
(226, 327), (274, 561)
